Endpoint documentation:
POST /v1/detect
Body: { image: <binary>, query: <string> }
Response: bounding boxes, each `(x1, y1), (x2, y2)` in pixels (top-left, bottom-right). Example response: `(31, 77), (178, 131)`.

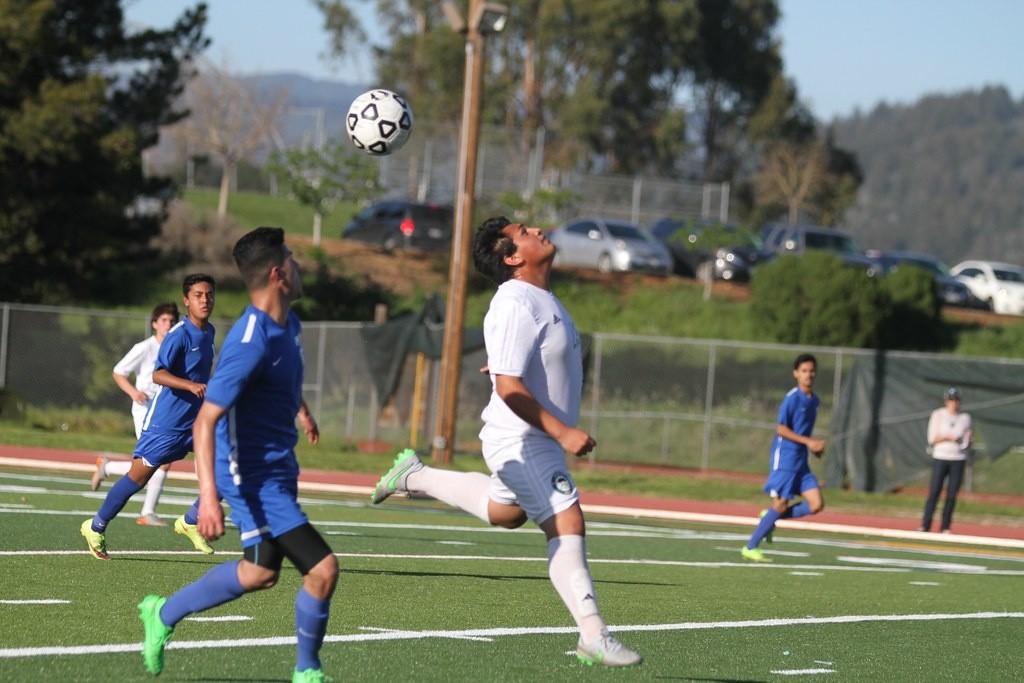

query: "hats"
(944, 387), (963, 399)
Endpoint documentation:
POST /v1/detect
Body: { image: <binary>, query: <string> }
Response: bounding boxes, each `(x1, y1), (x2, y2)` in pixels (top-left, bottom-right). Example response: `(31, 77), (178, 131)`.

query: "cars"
(865, 249), (972, 308)
(129, 191), (171, 223)
(947, 260), (1024, 318)
(545, 215), (675, 279)
(342, 195), (457, 255)
(758, 219), (875, 288)
(648, 214), (779, 289)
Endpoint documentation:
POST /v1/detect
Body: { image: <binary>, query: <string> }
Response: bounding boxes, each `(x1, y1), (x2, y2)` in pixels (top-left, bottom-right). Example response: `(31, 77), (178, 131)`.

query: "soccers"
(345, 88), (414, 157)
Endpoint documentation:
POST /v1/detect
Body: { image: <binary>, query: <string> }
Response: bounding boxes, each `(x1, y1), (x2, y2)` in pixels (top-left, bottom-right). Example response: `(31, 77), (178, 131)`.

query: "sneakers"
(80, 518), (111, 560)
(292, 667), (327, 683)
(576, 627), (641, 666)
(137, 594), (173, 675)
(371, 448), (421, 505)
(174, 514), (214, 555)
(90, 454), (111, 491)
(758, 510), (774, 543)
(136, 514), (168, 527)
(741, 545), (773, 563)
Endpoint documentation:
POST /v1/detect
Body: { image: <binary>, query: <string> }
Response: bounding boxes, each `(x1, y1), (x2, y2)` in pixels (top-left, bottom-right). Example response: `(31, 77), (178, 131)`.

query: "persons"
(136, 227), (339, 683)
(88, 302), (181, 526)
(920, 389), (972, 535)
(740, 354), (825, 563)
(370, 217), (643, 671)
(80, 275), (225, 561)
